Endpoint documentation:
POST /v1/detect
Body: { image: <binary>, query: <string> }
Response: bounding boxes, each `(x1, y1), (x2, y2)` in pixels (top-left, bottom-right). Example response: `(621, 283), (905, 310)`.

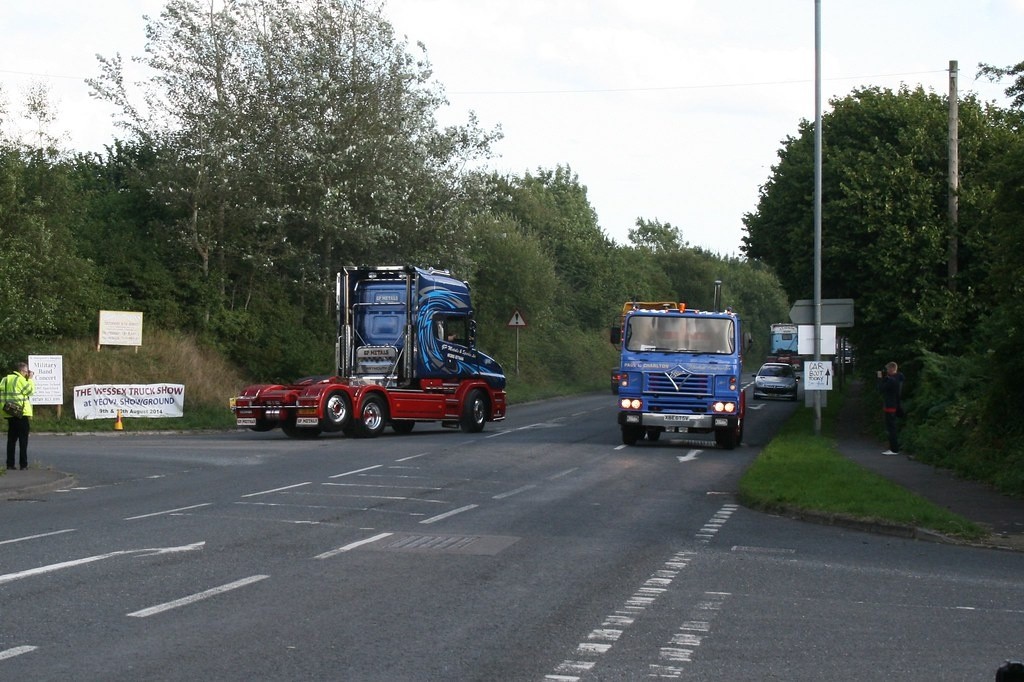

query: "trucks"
(769, 323), (798, 354)
(610, 299), (677, 391)
(609, 301), (748, 450)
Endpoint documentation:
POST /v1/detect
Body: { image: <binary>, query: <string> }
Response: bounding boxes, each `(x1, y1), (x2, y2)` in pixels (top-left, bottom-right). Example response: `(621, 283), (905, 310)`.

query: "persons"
(877, 362), (901, 456)
(0, 362), (35, 471)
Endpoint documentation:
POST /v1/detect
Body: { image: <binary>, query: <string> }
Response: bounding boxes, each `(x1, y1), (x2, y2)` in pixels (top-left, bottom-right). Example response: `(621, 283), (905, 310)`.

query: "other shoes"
(882, 449), (899, 455)
(7, 466), (17, 470)
(20, 467), (27, 470)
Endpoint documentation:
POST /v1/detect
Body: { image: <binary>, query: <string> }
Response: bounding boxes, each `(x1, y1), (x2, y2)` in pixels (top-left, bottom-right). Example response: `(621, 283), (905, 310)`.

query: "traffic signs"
(804, 361), (834, 391)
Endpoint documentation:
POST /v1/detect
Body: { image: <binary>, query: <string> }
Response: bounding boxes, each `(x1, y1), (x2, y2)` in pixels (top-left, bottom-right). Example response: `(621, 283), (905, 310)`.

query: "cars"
(766, 354), (802, 377)
(752, 362), (801, 401)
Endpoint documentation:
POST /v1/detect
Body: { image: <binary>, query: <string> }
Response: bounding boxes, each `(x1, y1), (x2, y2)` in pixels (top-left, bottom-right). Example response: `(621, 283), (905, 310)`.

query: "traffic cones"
(114, 409), (125, 432)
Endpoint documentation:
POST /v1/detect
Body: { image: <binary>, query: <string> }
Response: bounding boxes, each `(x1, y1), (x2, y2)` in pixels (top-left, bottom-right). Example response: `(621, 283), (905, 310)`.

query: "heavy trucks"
(229, 263), (508, 434)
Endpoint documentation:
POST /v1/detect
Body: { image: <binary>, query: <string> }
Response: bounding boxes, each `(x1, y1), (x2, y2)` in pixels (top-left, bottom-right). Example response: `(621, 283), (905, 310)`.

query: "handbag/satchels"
(3, 402), (23, 419)
